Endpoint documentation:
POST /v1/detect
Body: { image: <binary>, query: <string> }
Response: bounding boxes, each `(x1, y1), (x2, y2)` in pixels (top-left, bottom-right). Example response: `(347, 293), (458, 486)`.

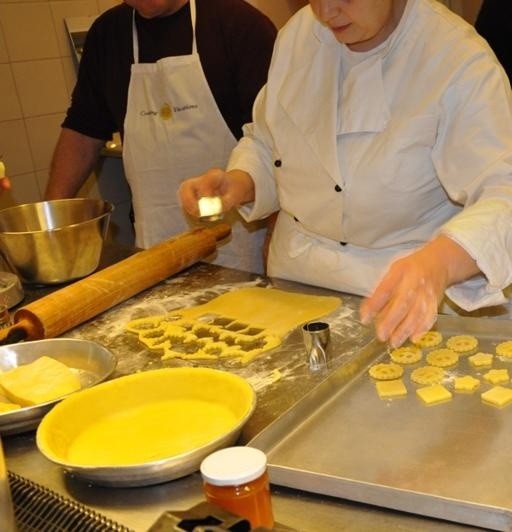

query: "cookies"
(370, 332), (512, 407)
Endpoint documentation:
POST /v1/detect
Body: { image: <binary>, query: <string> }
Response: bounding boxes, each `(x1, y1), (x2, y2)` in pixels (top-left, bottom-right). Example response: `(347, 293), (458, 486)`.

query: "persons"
(176, 0), (511, 349)
(43, 0), (278, 278)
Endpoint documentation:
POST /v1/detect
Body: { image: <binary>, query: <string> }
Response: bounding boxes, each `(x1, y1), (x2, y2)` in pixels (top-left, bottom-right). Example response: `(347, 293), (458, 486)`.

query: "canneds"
(200, 446), (275, 530)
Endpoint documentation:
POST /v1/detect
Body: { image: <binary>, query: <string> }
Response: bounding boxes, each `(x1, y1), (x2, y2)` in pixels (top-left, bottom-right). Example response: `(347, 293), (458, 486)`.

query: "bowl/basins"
(0, 272), (24, 310)
(33, 367), (254, 490)
(0, 196), (116, 291)
(0, 335), (116, 438)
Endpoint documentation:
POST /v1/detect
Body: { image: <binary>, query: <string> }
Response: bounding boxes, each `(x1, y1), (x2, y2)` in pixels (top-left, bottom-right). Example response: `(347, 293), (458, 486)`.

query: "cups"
(304, 322), (331, 365)
